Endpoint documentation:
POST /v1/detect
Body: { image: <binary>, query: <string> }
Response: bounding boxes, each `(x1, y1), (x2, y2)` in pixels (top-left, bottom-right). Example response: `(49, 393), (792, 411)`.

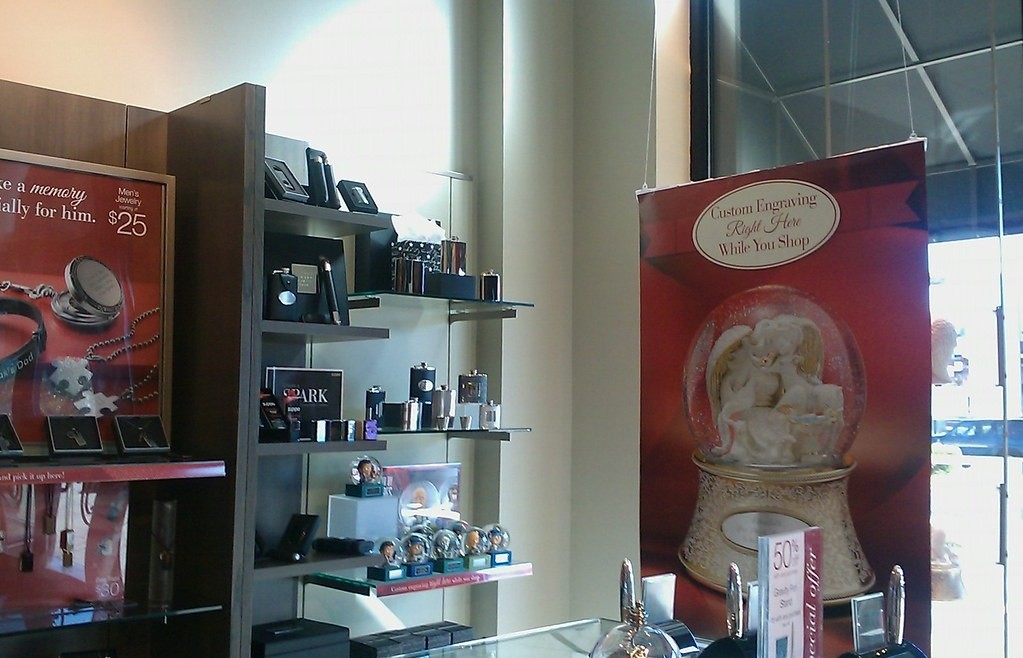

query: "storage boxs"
(365, 564), (406, 582)
(351, 634), (388, 658)
(411, 628), (453, 649)
(43, 414), (108, 458)
(377, 629), (410, 638)
(363, 639), (401, 658)
(0, 411), (26, 459)
(404, 560), (437, 577)
(112, 411), (173, 458)
(337, 177), (380, 212)
(436, 556), (462, 573)
(428, 620), (460, 629)
(265, 365), (344, 441)
(407, 625), (435, 634)
(390, 634), (426, 654)
(265, 155), (312, 201)
(260, 387), (287, 433)
(443, 624), (475, 644)
(251, 613), (355, 658)
(464, 554), (492, 567)
(489, 549), (513, 565)
(480, 273), (501, 302)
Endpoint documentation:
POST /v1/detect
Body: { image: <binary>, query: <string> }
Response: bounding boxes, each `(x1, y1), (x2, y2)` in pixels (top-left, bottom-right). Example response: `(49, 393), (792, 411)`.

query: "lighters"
(480, 269), (500, 302)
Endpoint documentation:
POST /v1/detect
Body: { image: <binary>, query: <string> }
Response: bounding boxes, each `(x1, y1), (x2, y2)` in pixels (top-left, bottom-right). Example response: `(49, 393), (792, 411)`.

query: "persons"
(380, 541), (400, 568)
(357, 460), (373, 482)
(405, 524), (502, 562)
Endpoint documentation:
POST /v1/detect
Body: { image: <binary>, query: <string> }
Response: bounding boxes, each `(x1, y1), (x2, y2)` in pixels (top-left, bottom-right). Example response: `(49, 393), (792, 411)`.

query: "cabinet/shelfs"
(242, 84), (396, 658)
(0, 74), (237, 658)
(309, 289), (536, 599)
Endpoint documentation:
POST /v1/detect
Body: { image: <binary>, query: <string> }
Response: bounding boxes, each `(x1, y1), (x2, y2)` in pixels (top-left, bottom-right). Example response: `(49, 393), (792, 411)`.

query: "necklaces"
(52, 307), (161, 418)
(18, 483), (73, 572)
